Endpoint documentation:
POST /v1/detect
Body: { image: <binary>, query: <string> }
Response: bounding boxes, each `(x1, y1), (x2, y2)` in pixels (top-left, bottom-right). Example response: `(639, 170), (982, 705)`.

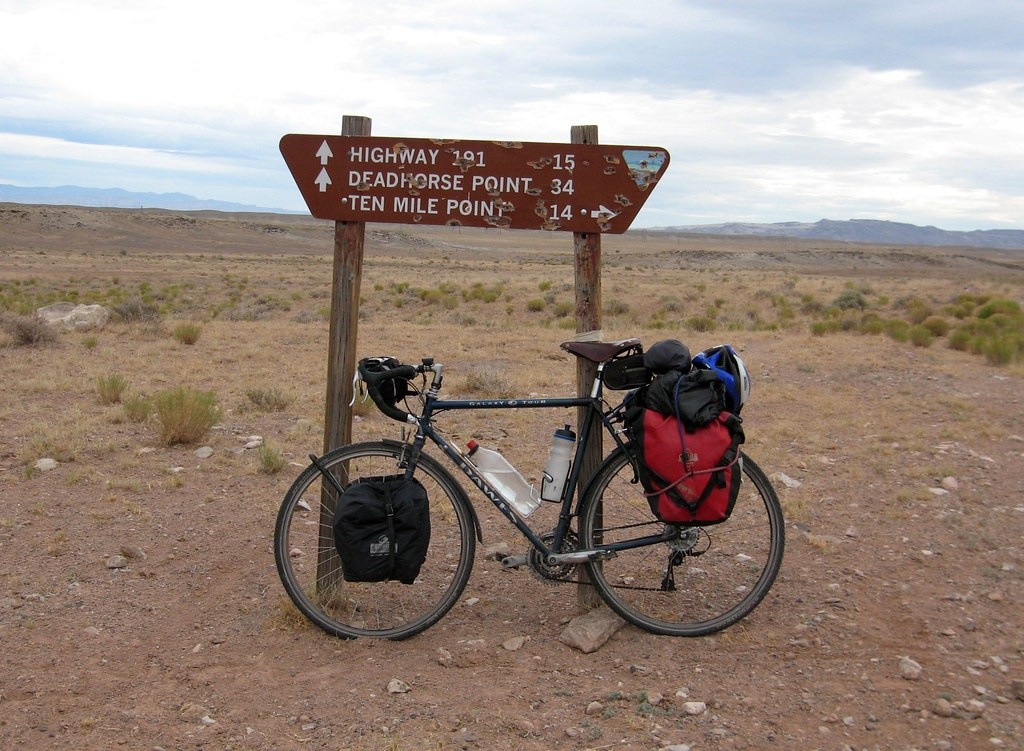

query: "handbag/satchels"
(626, 406), (746, 526)
(333, 475), (431, 585)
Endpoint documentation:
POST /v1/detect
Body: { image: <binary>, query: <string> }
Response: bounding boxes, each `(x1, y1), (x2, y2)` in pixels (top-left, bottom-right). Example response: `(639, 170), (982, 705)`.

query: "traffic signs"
(279, 133), (670, 234)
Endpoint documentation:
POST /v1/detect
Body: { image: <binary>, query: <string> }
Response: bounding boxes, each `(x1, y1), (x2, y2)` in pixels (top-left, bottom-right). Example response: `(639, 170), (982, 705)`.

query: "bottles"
(541, 423), (578, 503)
(465, 441), (541, 516)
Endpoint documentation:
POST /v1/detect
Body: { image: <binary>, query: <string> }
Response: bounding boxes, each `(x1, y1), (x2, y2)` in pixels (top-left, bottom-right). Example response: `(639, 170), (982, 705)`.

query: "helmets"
(691, 344), (751, 416)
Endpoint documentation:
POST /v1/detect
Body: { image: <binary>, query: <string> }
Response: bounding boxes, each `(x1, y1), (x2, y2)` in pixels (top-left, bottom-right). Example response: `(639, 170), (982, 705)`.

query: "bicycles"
(273, 336), (785, 638)
